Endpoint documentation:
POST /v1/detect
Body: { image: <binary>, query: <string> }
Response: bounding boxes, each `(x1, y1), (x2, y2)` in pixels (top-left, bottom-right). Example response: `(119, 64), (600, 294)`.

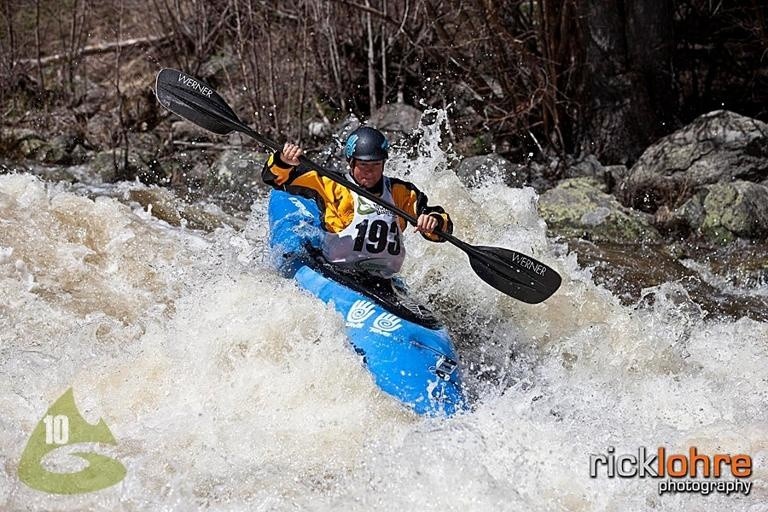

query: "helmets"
(344, 127), (388, 162)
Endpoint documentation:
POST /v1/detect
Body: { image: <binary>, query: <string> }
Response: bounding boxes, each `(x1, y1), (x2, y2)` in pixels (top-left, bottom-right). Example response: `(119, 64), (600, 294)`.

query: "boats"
(268, 188), (471, 418)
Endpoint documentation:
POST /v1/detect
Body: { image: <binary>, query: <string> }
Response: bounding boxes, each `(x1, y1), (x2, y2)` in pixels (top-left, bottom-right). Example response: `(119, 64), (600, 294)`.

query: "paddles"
(156, 68), (561, 304)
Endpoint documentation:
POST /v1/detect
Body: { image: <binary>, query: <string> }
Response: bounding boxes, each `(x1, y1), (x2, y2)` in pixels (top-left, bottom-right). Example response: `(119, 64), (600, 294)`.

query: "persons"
(261, 126), (453, 307)
